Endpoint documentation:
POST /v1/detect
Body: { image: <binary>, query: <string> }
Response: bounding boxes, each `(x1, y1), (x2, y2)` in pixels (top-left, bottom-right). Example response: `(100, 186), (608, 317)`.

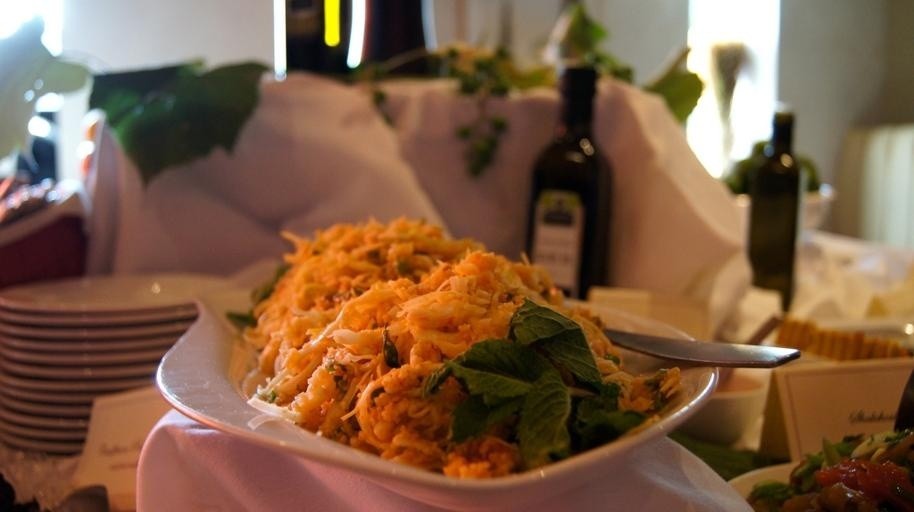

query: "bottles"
(522, 63), (614, 299)
(748, 111), (799, 314)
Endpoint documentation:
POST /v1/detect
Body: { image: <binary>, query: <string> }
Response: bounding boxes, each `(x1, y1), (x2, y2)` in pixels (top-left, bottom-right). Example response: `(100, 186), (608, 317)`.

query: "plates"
(728, 457), (809, 512)
(0, 273), (228, 455)
(158, 261), (720, 511)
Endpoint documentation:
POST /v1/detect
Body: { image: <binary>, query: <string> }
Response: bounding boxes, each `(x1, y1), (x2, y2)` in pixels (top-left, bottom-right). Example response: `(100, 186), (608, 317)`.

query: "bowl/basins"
(737, 187), (833, 241)
(684, 368), (770, 446)
